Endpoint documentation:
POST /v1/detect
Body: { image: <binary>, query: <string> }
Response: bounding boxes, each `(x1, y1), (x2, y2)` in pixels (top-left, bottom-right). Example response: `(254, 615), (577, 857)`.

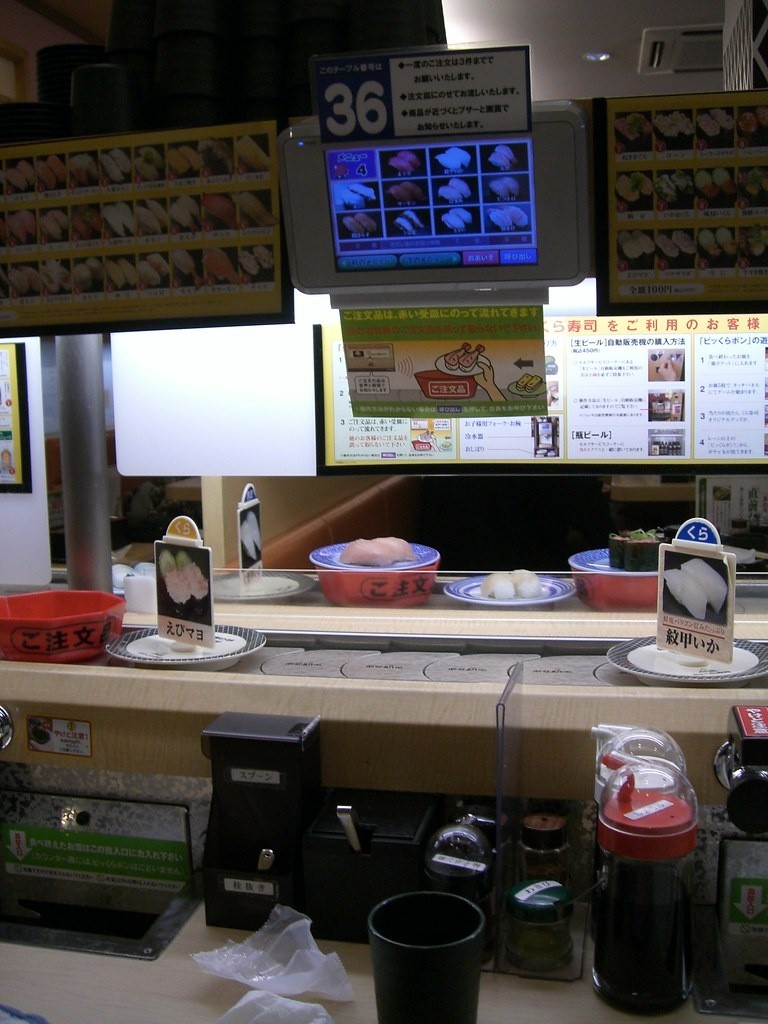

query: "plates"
(443, 574), (577, 606)
(309, 542), (440, 571)
(606, 636), (768, 687)
(105, 625), (267, 671)
(568, 548), (659, 576)
(211, 569), (315, 603)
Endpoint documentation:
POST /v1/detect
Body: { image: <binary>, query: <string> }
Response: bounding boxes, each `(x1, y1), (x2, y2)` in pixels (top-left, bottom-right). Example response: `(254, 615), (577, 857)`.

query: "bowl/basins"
(0, 588), (127, 662)
(313, 561), (440, 607)
(570, 567), (658, 611)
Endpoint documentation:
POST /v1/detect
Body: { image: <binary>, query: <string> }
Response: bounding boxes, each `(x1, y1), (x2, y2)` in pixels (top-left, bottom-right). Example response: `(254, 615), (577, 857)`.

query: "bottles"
(505, 879), (573, 971)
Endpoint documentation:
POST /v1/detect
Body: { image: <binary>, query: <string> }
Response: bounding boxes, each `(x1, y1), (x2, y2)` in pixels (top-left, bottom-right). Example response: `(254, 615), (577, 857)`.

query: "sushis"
(612, 105), (768, 270)
(608, 529), (681, 571)
(158, 548), (208, 604)
(0, 135), (275, 293)
(342, 145), (528, 235)
(483, 568), (544, 601)
(339, 537), (413, 567)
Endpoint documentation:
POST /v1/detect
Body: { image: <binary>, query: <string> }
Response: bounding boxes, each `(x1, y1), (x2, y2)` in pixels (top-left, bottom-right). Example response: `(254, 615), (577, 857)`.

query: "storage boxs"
(301, 788), (443, 944)
(197, 710), (321, 869)
(201, 869), (303, 931)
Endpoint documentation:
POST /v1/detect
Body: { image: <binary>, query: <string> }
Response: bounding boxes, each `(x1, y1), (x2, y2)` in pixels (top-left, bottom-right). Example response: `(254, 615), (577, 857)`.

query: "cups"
(370, 891), (485, 1024)
(592, 731), (695, 1016)
